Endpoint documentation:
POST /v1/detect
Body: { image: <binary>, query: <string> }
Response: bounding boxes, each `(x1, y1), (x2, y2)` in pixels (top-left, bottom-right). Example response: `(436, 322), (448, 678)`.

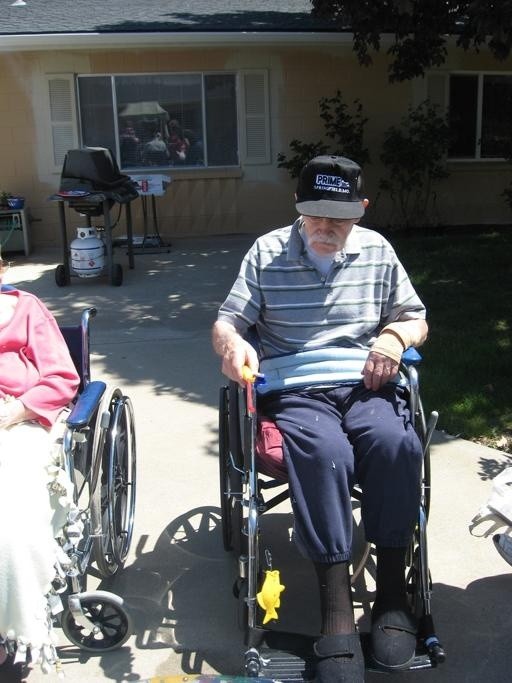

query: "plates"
(56, 190), (92, 197)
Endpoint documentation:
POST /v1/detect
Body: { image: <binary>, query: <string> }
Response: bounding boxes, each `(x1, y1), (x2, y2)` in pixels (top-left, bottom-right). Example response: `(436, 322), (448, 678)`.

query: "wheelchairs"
(215, 324), (447, 680)
(141, 146), (169, 167)
(1, 305), (137, 651)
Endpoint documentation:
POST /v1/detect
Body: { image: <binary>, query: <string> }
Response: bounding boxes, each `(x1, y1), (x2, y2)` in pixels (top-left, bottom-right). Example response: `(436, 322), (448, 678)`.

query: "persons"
(0, 256), (82, 680)
(119, 118), (204, 166)
(211, 154), (429, 683)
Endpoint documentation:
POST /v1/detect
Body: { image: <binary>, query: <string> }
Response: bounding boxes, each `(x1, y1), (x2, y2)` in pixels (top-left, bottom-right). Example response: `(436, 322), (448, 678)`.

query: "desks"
(0, 206), (34, 258)
(135, 178), (172, 250)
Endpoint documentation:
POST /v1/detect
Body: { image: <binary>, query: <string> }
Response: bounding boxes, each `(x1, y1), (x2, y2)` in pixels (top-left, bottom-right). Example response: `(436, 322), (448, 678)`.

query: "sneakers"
(314, 624), (364, 682)
(368, 598), (419, 671)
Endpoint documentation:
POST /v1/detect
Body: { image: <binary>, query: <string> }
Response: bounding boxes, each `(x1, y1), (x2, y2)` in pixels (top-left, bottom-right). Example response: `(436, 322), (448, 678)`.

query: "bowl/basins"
(7, 197), (24, 208)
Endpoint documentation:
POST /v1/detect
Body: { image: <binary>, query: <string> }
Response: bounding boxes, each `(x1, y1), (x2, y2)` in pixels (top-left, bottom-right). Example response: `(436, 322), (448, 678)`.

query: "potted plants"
(0, 191), (14, 209)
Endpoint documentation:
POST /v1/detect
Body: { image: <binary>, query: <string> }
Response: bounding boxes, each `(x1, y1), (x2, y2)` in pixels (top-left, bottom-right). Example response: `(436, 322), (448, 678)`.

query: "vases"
(7, 196), (25, 209)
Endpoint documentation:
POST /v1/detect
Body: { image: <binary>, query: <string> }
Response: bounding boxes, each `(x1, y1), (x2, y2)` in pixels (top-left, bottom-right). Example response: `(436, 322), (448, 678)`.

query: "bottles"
(142, 179), (148, 191)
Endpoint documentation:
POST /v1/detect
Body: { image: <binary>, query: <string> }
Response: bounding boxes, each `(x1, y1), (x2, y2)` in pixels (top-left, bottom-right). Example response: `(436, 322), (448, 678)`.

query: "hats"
(295, 155), (365, 220)
